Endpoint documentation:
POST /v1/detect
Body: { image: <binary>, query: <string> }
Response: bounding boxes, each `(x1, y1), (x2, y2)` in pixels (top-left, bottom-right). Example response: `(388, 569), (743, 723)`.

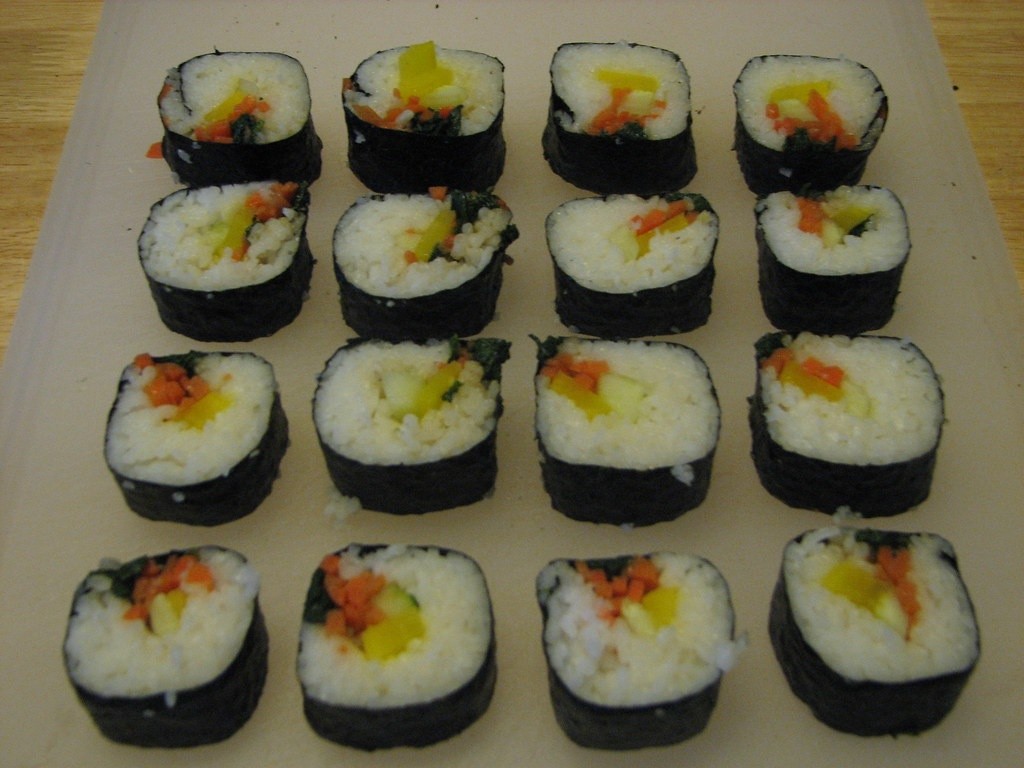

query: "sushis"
(60, 38), (983, 755)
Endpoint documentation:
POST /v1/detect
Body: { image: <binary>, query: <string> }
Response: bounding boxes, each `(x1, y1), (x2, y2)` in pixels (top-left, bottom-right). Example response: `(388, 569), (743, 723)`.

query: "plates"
(0, 0), (1024, 768)
